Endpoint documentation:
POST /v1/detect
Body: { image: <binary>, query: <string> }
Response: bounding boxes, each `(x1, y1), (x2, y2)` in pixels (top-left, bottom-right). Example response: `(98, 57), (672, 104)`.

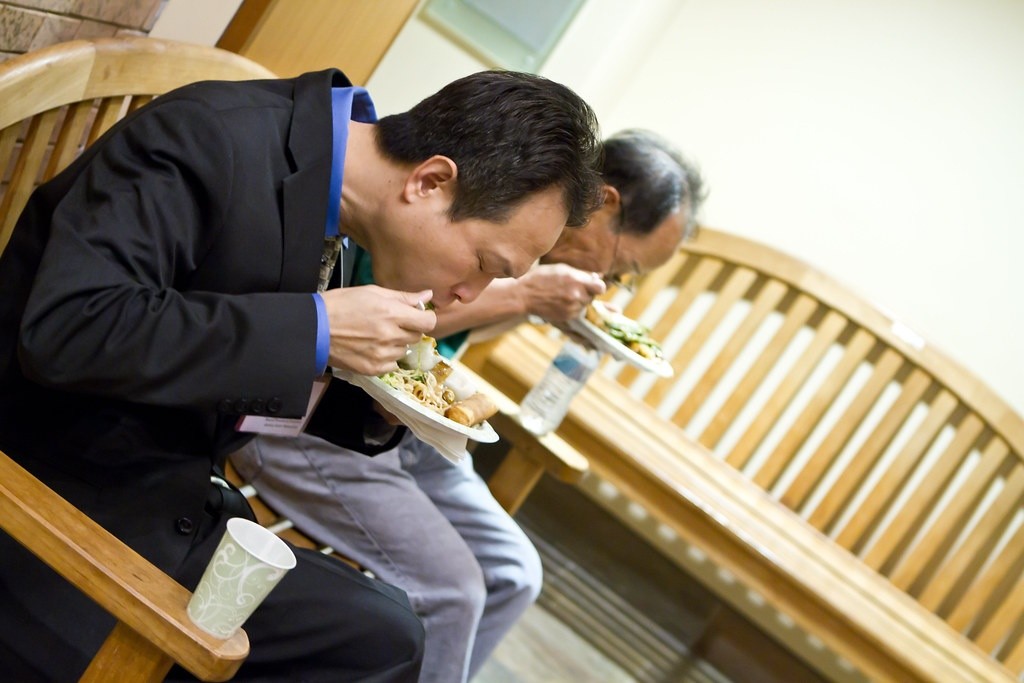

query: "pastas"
(381, 367), (450, 414)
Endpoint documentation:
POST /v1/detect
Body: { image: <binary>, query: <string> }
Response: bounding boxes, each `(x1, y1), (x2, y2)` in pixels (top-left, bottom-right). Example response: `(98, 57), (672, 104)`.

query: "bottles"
(520, 339), (603, 435)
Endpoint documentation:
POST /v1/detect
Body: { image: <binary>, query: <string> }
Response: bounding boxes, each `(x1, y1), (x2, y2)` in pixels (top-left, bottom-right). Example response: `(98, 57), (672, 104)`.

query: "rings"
(405, 347), (411, 360)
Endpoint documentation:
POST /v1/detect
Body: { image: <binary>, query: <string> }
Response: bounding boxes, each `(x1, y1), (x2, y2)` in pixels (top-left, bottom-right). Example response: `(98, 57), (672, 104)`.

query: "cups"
(185, 518), (297, 639)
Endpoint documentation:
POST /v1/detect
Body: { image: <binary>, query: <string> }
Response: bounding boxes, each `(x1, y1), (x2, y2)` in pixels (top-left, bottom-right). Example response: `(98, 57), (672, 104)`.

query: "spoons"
(401, 300), (442, 371)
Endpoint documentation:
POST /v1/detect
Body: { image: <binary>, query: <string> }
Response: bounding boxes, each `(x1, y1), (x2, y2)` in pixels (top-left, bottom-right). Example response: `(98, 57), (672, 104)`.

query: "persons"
(228, 134), (706, 681)
(0, 65), (600, 683)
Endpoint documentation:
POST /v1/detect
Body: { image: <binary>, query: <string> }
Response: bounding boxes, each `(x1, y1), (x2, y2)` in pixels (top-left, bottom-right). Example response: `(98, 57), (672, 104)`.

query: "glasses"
(603, 199), (633, 296)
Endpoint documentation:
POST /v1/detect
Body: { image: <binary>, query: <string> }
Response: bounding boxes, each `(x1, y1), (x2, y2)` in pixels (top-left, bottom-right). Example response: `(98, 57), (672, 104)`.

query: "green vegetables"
(602, 320), (658, 348)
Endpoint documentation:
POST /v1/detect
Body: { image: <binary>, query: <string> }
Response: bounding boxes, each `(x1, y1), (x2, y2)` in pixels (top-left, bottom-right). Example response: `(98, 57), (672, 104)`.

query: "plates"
(364, 375), (499, 443)
(566, 301), (675, 378)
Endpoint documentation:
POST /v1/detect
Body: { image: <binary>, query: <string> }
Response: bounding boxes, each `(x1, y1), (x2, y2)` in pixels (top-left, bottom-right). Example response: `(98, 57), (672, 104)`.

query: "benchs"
(456, 227), (1024, 683)
(0, 37), (589, 683)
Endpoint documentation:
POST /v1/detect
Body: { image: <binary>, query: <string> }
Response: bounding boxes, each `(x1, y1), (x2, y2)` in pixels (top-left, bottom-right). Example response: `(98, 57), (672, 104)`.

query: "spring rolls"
(444, 392), (500, 426)
